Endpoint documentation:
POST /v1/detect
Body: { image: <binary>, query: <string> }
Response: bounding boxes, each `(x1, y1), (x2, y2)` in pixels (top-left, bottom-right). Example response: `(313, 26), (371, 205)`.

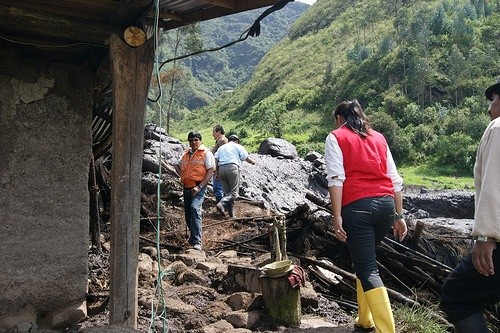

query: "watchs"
(475, 235), (494, 243)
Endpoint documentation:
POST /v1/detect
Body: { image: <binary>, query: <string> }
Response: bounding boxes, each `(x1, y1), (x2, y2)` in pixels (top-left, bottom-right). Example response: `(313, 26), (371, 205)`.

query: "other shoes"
(216, 203), (226, 216)
(194, 244), (201, 250)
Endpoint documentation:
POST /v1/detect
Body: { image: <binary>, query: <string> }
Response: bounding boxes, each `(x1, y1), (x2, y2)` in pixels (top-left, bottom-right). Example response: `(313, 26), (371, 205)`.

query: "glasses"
(189, 139), (199, 142)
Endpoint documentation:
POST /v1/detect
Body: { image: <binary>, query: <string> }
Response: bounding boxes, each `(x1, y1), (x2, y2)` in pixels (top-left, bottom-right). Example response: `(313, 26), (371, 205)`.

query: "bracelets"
(199, 184), (203, 189)
(394, 213), (405, 222)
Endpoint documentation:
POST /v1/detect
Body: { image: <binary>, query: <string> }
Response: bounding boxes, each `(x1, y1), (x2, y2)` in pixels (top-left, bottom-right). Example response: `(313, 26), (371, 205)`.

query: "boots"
(354, 277), (375, 330)
(365, 287), (395, 333)
(216, 196), (223, 214)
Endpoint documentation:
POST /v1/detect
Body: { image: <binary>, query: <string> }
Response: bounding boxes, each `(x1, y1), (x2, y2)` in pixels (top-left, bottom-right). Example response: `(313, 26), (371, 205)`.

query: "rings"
(337, 230), (340, 233)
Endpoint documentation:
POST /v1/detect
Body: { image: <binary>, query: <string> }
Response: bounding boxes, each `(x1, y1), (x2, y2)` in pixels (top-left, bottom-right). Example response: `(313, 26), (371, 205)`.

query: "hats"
(228, 135), (239, 143)
(485, 75), (500, 101)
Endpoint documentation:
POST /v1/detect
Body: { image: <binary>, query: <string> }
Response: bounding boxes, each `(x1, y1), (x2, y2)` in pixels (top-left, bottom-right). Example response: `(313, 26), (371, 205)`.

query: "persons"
(438, 76), (500, 333)
(214, 135), (256, 217)
(173, 132), (214, 251)
(323, 98), (408, 333)
(211, 124), (229, 215)
